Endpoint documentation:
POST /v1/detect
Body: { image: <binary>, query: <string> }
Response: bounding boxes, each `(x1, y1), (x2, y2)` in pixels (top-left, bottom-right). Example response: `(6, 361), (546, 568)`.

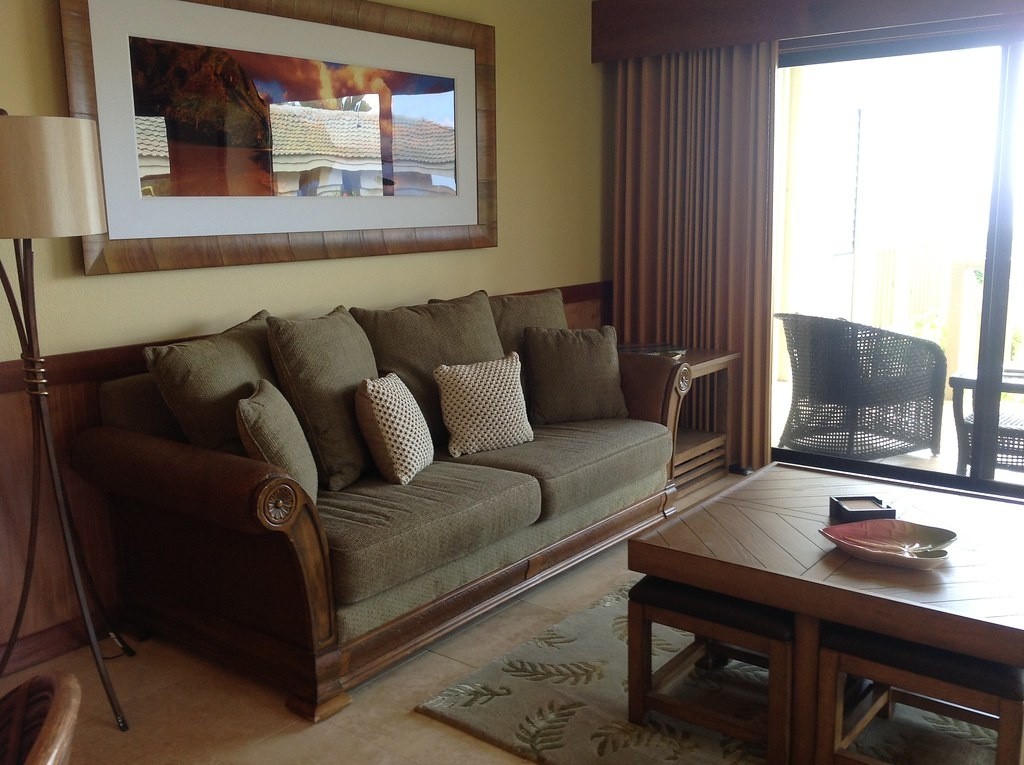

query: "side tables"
(675, 346), (742, 499)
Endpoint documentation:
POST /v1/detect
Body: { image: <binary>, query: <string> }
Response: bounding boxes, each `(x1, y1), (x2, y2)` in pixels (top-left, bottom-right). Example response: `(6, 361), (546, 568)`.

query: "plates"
(818, 519), (957, 572)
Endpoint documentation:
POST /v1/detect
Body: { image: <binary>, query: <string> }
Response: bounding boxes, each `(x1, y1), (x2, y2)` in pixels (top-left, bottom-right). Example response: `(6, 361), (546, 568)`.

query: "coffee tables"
(627, 463), (1024, 765)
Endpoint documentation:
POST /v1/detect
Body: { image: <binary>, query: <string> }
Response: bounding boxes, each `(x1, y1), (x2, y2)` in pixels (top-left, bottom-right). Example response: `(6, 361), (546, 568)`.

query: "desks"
(949, 367), (1024, 476)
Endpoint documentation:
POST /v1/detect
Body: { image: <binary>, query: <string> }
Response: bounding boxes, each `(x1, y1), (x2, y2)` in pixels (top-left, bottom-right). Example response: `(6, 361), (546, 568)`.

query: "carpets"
(413, 572), (998, 765)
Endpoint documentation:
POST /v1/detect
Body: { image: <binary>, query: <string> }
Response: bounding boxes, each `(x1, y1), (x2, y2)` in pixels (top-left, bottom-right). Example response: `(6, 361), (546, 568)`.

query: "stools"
(628, 575), (794, 765)
(813, 625), (1024, 765)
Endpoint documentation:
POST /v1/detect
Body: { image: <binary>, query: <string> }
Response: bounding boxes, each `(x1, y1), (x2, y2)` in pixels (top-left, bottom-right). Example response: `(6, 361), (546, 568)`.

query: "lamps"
(0, 109), (137, 734)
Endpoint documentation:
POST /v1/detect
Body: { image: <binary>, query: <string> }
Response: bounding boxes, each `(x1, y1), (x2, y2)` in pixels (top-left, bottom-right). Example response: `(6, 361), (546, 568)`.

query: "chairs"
(773, 311), (947, 461)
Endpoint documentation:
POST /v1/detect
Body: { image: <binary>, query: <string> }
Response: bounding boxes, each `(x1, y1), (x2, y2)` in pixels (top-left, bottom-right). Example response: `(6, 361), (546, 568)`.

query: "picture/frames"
(56, 0), (499, 276)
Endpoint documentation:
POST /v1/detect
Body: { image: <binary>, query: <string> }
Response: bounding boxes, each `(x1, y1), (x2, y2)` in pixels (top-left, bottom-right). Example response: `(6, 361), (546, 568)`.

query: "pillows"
(524, 325), (630, 426)
(266, 304), (379, 493)
(349, 290), (505, 443)
(428, 288), (568, 417)
(141, 309), (280, 457)
(432, 351), (535, 459)
(235, 379), (319, 505)
(354, 372), (434, 486)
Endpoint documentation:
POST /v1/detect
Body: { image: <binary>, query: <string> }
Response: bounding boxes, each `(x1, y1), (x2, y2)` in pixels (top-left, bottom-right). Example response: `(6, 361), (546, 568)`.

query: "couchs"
(64, 352), (694, 726)
